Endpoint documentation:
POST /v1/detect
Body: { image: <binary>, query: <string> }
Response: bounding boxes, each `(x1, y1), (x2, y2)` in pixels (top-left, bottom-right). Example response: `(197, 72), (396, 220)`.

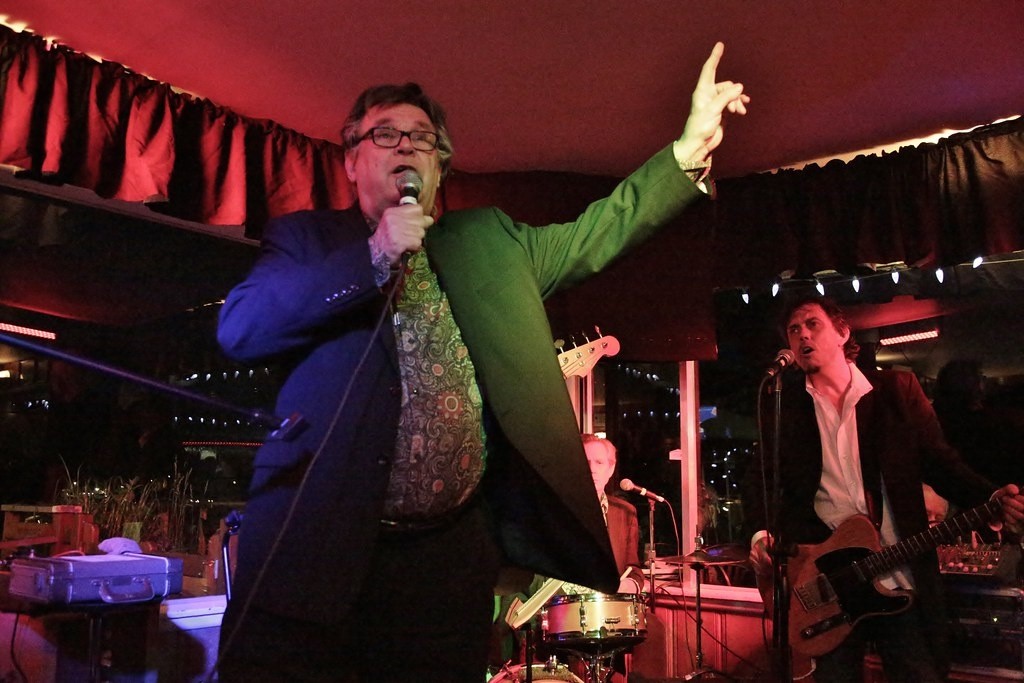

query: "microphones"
(396, 170), (423, 259)
(765, 349), (795, 379)
(620, 479), (668, 504)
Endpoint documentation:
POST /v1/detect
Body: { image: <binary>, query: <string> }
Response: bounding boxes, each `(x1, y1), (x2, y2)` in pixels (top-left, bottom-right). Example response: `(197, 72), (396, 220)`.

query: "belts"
(379, 490), (477, 538)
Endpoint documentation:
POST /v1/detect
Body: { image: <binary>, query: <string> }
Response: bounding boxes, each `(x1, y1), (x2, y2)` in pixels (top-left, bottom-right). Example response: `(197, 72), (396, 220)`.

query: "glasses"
(352, 127), (441, 152)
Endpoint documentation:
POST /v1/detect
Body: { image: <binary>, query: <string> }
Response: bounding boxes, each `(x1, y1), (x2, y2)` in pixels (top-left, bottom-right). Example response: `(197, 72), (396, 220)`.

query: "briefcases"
(9, 552), (182, 605)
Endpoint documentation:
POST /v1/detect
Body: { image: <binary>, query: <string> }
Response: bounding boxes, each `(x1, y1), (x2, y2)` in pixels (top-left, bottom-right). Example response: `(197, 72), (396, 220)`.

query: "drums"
(493, 657), (629, 682)
(541, 591), (651, 643)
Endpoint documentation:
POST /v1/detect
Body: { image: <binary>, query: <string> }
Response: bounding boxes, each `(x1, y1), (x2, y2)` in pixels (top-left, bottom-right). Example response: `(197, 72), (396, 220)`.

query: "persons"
(210, 40), (750, 682)
(517, 432), (645, 683)
(747, 292), (1024, 683)
(654, 437), (682, 498)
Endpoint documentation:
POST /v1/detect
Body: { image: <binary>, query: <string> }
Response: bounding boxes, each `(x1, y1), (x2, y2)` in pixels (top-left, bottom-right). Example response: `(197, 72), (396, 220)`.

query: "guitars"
(759, 488), (1023, 653)
(556, 328), (621, 379)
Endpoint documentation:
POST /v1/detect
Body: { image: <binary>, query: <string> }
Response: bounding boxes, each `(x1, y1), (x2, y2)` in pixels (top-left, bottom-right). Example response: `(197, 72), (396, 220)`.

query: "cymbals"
(654, 554), (747, 568)
